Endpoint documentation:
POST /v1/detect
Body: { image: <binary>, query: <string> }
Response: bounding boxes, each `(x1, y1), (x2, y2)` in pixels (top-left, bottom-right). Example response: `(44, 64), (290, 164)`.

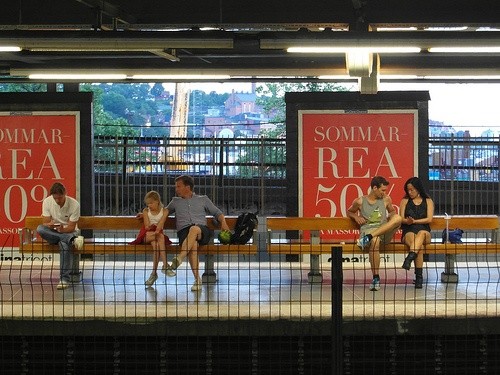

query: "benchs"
(18, 215), (500, 285)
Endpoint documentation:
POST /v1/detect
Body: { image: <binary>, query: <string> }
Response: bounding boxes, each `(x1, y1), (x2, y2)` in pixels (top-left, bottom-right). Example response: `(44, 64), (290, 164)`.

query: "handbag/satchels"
(231, 213), (258, 245)
(442, 228), (463, 244)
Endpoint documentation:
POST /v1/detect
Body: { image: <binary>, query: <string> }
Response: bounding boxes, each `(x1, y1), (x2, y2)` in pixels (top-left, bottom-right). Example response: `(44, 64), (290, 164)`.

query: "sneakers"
(370, 278), (380, 291)
(356, 236), (369, 251)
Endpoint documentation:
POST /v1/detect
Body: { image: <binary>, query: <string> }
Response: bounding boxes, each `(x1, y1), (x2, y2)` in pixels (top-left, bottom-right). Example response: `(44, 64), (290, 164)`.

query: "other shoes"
(171, 257), (182, 270)
(74, 236), (84, 250)
(162, 264), (176, 277)
(416, 276), (423, 288)
(57, 280), (70, 289)
(191, 281), (202, 291)
(402, 257), (412, 270)
(145, 274), (157, 286)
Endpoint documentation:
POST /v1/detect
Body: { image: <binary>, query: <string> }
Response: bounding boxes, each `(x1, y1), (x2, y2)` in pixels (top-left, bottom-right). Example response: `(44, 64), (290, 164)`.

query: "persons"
(347, 176), (402, 291)
(37, 183), (85, 290)
(142, 191), (176, 287)
(136, 175), (231, 291)
(400, 177), (434, 288)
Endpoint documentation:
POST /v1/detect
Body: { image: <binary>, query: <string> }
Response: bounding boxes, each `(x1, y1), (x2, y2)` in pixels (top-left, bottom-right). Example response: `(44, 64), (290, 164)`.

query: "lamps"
(283, 47), (422, 54)
(427, 47), (500, 53)
(0, 47), (23, 52)
